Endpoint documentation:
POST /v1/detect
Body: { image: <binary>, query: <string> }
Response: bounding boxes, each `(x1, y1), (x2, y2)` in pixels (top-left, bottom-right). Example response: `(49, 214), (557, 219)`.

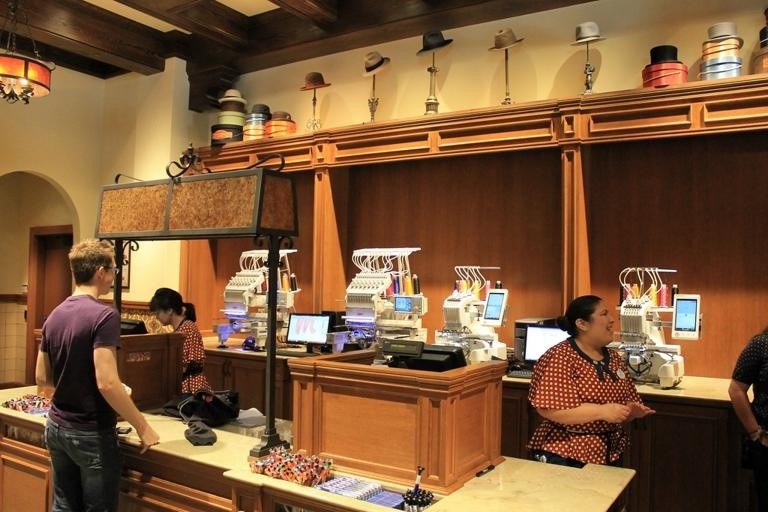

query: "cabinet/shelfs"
(500, 384), (768, 512)
(202, 350), (289, 420)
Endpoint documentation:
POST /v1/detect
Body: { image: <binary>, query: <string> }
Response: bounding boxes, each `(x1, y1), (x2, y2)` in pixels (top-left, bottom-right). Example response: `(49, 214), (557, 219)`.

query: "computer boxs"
(514, 318), (556, 369)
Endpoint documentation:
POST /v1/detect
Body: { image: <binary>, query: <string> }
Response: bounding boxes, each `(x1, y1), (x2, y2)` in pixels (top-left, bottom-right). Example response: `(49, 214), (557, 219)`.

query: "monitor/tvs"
(120, 317), (147, 334)
(524, 324), (572, 363)
(393, 343), (467, 372)
(287, 313), (330, 352)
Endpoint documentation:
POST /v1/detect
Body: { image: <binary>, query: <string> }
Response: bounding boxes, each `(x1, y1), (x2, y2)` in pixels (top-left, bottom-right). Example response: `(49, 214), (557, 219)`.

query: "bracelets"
(749, 425), (760, 435)
(752, 429), (768, 442)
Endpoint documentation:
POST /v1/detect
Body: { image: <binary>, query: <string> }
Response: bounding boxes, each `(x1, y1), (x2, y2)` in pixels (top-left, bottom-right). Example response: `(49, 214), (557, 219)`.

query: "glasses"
(104, 267), (119, 275)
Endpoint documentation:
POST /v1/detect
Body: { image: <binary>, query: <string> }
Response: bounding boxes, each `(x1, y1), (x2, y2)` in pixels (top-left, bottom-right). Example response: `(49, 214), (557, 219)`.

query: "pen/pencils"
(402, 465), (433, 512)
(249, 445), (334, 487)
(476, 464), (494, 478)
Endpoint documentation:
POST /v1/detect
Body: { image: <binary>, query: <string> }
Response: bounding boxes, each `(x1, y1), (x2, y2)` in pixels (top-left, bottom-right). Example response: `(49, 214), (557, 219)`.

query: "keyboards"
(276, 349), (319, 357)
(506, 369), (535, 379)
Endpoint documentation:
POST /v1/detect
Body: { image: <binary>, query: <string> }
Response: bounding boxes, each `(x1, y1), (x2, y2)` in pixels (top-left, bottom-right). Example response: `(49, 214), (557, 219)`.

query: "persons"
(147, 287), (212, 398)
(523, 294), (657, 470)
(729, 326), (768, 511)
(35, 239), (161, 512)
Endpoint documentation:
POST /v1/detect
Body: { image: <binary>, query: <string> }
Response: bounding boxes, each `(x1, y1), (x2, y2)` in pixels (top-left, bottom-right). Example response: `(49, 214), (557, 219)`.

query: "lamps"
(0, 0), (56, 105)
